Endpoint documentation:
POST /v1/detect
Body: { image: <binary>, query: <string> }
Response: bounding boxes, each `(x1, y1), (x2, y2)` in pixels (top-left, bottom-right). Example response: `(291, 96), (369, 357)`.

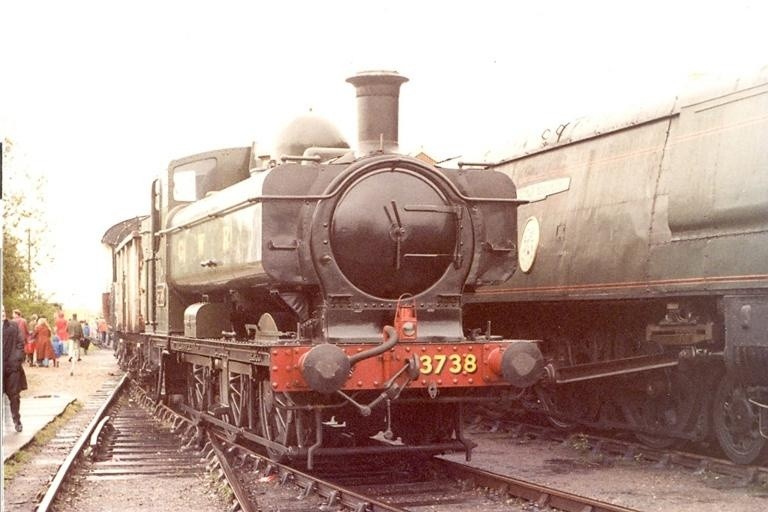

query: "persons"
(13, 308), (114, 368)
(2, 302), (29, 433)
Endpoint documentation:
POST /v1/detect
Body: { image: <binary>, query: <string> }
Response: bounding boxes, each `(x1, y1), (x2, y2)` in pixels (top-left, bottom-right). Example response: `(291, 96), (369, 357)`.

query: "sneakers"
(13, 419), (23, 434)
